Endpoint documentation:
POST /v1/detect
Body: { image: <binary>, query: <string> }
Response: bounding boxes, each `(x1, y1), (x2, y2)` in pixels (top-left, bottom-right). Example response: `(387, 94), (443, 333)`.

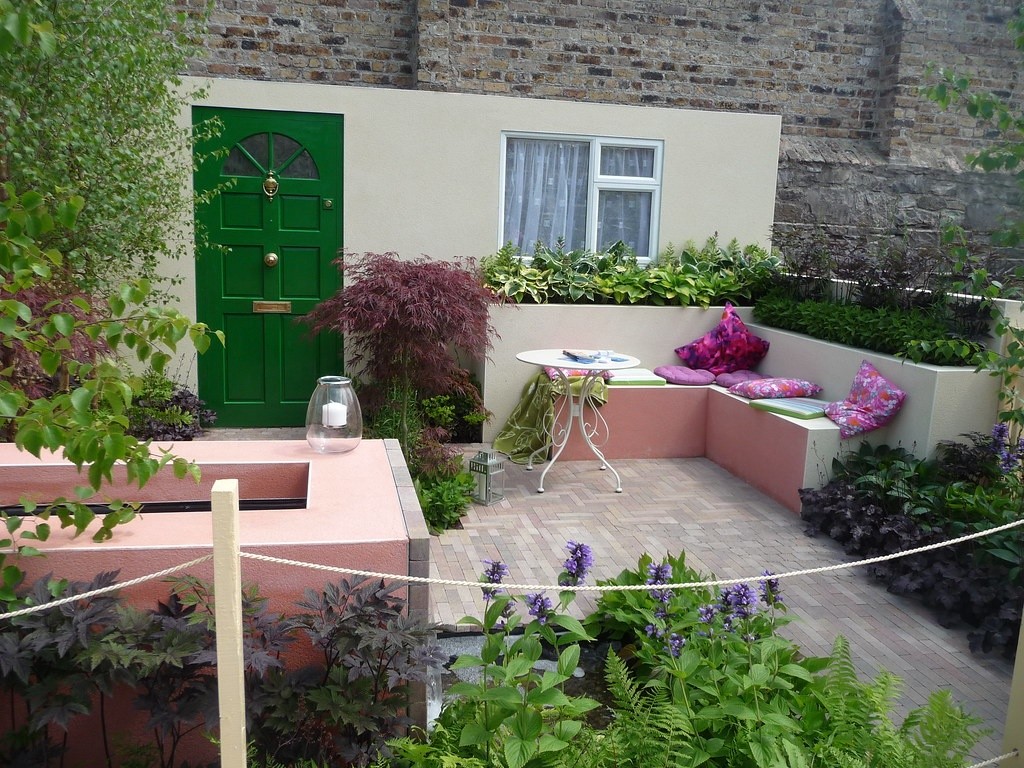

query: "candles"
(322, 399), (347, 427)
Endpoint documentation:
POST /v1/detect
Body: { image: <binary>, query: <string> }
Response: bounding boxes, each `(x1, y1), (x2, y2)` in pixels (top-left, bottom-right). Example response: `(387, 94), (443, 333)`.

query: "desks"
(515, 349), (641, 494)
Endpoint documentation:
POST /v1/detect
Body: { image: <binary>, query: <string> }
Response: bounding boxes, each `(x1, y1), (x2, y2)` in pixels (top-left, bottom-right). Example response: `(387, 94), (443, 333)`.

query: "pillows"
(823, 359), (906, 439)
(544, 366), (614, 381)
(675, 302), (771, 376)
(726, 376), (822, 399)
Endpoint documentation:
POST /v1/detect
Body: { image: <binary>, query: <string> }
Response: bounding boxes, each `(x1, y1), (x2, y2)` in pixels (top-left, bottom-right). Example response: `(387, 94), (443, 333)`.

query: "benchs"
(549, 384), (887, 520)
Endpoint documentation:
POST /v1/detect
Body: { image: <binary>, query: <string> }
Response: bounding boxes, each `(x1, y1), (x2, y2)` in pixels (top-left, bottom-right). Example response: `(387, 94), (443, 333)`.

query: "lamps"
(468, 448), (507, 506)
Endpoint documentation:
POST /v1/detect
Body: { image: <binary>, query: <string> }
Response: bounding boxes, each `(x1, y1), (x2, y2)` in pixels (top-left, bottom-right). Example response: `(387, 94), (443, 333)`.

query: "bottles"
(305, 375), (363, 454)
